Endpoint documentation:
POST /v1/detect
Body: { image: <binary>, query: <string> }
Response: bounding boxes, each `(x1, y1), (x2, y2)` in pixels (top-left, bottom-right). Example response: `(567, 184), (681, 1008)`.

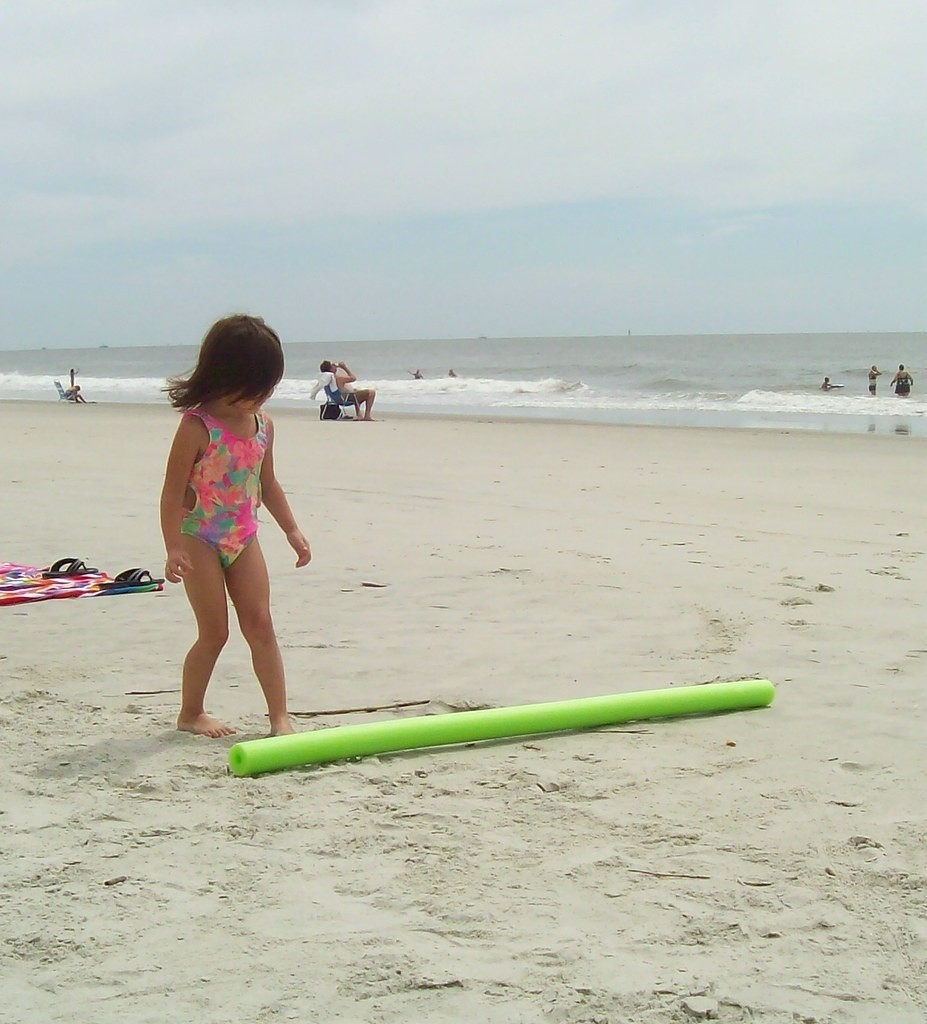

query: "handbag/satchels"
(320, 402), (343, 421)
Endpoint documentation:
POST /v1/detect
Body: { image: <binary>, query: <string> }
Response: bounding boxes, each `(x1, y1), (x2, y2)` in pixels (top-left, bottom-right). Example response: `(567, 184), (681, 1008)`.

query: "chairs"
(310, 372), (363, 421)
(54, 380), (76, 402)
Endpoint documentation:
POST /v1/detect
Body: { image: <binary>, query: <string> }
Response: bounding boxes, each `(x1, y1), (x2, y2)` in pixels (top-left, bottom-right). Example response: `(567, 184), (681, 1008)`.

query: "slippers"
(97, 567), (166, 591)
(41, 558), (98, 578)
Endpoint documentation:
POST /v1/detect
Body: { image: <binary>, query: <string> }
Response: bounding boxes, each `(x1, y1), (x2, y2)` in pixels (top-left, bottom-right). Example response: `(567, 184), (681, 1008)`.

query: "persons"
(448, 368), (458, 377)
(890, 364), (913, 397)
(408, 369), (424, 379)
(320, 361), (376, 422)
(868, 366), (882, 396)
(819, 377), (833, 391)
(66, 369), (87, 403)
(161, 314), (309, 738)
